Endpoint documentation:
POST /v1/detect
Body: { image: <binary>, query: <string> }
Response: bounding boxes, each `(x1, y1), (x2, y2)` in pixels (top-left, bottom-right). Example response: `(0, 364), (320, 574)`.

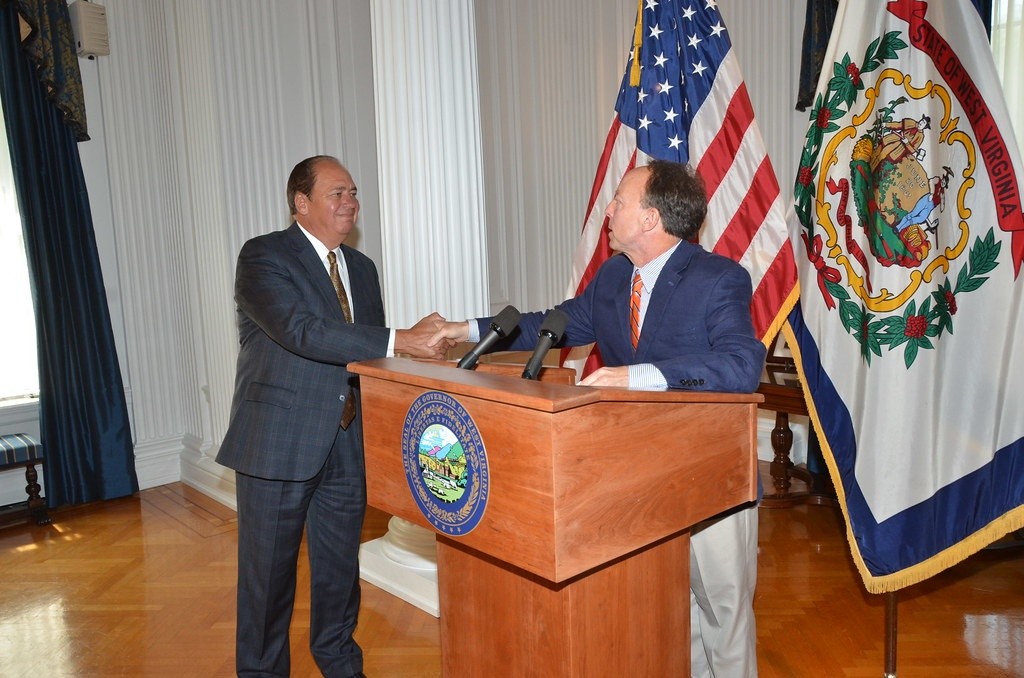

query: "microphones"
(521, 310), (569, 379)
(456, 305), (521, 370)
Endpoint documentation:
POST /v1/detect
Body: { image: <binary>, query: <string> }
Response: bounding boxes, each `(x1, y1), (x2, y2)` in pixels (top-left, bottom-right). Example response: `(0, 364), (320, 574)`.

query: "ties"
(630, 273), (644, 354)
(328, 251), (357, 431)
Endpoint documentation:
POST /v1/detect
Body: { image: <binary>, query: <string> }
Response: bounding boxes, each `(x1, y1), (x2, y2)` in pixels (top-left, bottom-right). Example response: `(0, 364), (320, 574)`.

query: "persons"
(211, 153), (456, 677)
(425, 157), (767, 678)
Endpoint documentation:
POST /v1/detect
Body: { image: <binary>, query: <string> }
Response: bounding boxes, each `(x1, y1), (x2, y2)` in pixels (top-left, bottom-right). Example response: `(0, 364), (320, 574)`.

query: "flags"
(561, 1), (1023, 600)
